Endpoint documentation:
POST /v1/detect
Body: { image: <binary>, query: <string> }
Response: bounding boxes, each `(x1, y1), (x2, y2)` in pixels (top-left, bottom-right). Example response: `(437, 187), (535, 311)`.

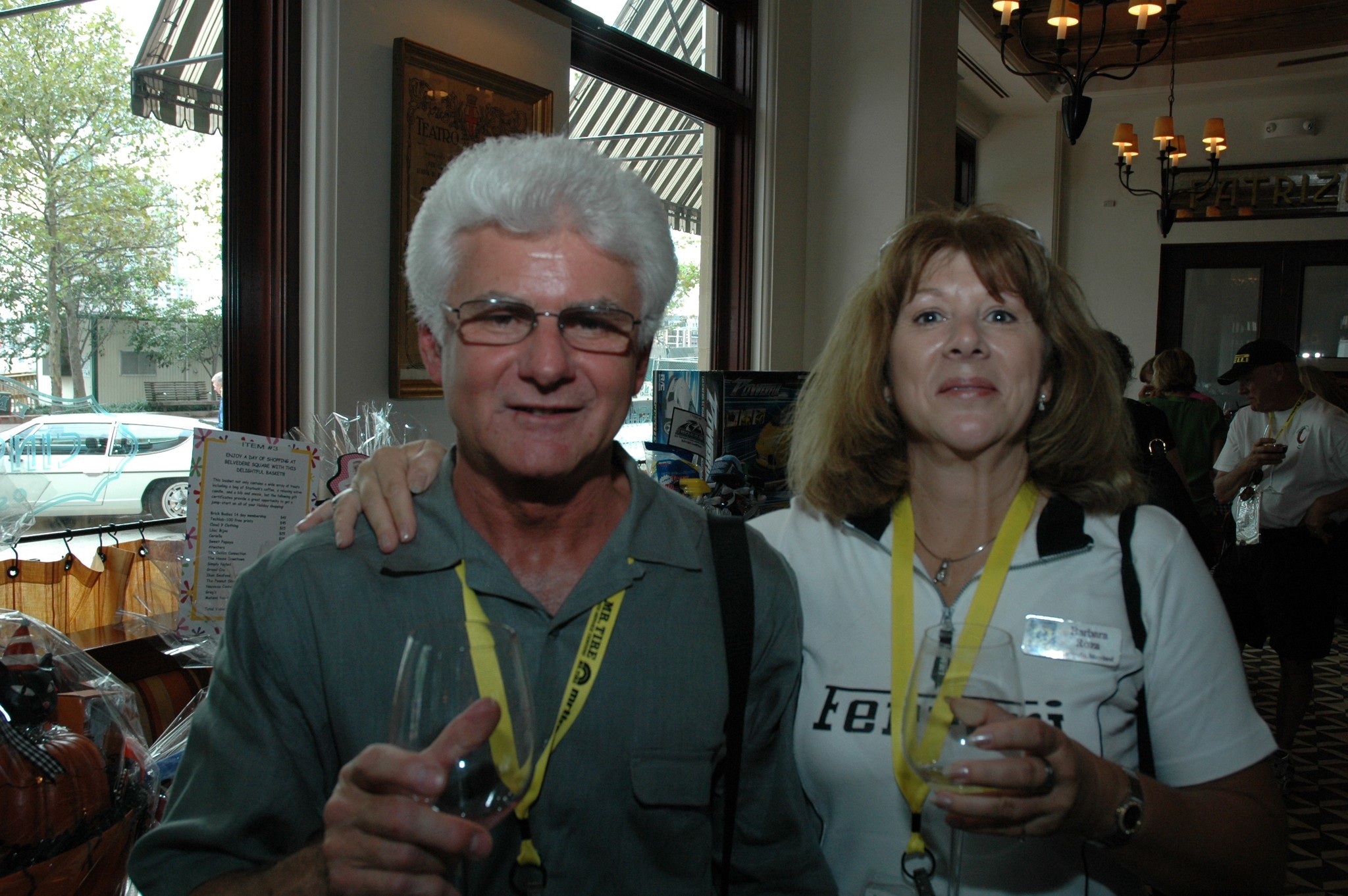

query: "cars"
(756, 400), (798, 477)
(0, 412), (224, 521)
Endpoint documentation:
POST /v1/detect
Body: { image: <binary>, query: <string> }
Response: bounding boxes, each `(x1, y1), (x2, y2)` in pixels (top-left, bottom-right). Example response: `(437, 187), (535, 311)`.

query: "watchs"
(1106, 765), (1146, 851)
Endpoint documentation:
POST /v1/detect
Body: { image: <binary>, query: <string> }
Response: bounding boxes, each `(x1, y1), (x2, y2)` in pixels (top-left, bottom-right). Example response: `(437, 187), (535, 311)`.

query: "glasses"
(438, 294), (642, 357)
(876, 215), (1052, 287)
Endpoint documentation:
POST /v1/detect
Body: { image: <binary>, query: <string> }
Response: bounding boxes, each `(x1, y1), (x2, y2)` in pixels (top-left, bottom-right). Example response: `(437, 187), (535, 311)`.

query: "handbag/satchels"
(1145, 438), (1204, 542)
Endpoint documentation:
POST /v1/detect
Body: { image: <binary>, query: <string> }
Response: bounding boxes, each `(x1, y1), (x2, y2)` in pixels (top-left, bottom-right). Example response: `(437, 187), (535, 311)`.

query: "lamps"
(1263, 118), (1316, 138)
(1112, 0), (1226, 237)
(991, 0), (1173, 146)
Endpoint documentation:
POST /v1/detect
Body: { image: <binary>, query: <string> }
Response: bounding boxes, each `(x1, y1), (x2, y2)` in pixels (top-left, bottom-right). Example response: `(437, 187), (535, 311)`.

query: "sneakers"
(1272, 749), (1295, 790)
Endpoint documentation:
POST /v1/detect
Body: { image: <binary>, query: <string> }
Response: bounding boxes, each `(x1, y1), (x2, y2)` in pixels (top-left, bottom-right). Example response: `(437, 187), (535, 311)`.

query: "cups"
(1223, 401), (1235, 421)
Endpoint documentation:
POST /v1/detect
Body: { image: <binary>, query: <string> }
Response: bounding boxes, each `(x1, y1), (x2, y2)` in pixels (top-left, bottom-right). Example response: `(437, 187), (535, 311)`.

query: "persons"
(1039, 322), (1232, 559)
(130, 125), (834, 896)
(1206, 337), (1348, 765)
(292, 204), (1284, 896)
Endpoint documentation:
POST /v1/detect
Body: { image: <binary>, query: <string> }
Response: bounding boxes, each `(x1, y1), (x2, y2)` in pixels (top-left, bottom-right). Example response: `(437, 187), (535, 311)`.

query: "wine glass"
(386, 618), (534, 894)
(899, 624), (1025, 896)
(1257, 424), (1289, 495)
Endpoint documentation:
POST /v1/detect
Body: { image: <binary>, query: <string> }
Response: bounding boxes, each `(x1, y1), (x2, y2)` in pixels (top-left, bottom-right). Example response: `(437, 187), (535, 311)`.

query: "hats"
(1216, 337), (1294, 385)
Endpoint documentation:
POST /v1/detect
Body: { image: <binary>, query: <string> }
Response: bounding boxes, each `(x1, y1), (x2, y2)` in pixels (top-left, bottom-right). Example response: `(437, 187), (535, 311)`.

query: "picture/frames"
(388, 36), (555, 401)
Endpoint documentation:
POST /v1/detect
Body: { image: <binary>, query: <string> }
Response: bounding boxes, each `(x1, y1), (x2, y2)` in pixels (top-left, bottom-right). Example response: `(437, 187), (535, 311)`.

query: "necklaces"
(912, 526), (998, 586)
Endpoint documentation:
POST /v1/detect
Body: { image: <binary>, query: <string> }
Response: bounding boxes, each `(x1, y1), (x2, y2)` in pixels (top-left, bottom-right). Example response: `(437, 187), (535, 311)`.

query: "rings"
(1037, 756), (1057, 792)
